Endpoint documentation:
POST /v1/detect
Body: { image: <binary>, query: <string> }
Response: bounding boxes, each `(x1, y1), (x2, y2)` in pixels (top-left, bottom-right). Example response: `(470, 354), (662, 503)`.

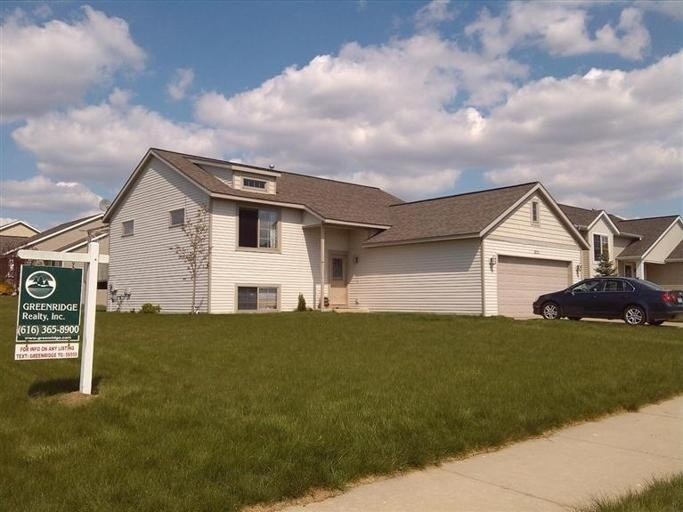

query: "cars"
(531, 275), (682, 324)
